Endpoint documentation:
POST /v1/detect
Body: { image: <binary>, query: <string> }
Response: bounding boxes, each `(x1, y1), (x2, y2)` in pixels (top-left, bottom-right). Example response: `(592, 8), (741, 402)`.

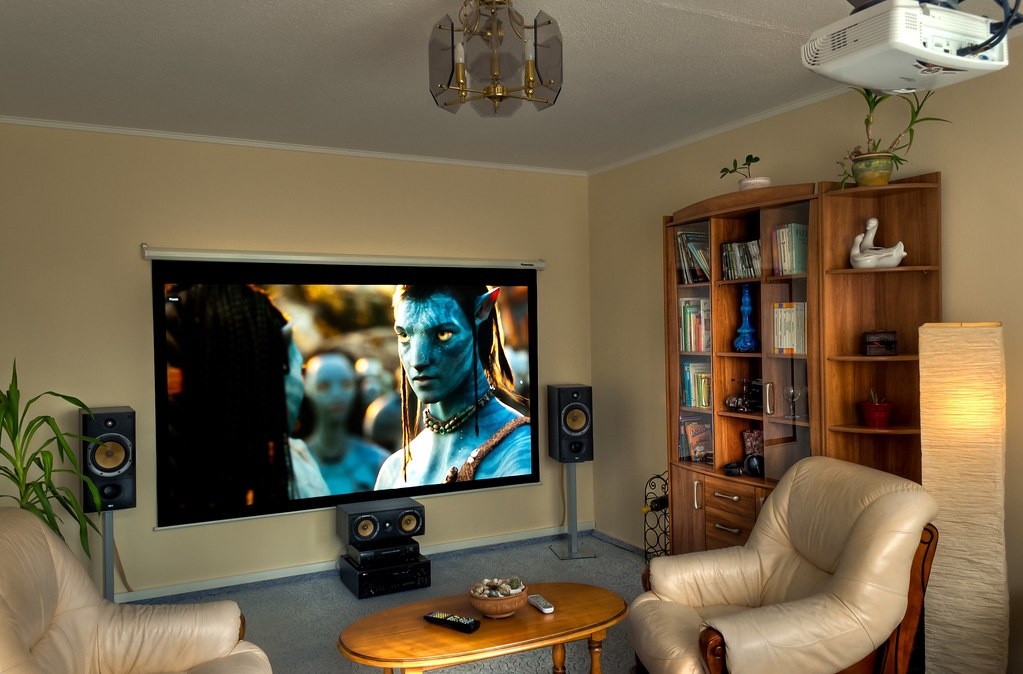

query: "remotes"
(528, 594), (555, 613)
(424, 612), (481, 635)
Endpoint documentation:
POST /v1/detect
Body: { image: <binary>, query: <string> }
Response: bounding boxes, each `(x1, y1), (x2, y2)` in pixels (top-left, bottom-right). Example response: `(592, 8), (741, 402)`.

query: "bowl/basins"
(469, 584), (527, 618)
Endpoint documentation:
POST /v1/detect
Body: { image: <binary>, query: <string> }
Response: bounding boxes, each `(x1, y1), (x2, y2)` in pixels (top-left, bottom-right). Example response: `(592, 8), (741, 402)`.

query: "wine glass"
(782, 384), (802, 419)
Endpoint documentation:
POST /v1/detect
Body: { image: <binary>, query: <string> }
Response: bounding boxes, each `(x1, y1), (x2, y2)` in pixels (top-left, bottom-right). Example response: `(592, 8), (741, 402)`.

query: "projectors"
(800, 0), (1008, 96)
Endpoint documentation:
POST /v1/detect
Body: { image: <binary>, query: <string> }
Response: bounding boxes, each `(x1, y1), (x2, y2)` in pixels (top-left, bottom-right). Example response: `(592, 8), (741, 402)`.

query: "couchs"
(627, 457), (940, 674)
(0, 506), (273, 674)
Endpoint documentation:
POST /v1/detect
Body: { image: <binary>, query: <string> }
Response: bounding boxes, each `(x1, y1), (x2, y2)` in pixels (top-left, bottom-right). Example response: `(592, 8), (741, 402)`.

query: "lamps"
(918, 322), (1011, 674)
(429, 0), (563, 118)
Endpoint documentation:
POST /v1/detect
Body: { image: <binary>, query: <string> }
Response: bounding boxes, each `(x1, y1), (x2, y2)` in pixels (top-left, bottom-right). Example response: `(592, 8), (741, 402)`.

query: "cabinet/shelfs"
(661, 172), (942, 550)
(644, 470), (669, 565)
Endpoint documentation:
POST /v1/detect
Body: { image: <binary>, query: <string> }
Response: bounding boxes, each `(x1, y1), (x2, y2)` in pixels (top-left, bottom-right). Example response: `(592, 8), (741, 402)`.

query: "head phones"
(724, 453), (765, 478)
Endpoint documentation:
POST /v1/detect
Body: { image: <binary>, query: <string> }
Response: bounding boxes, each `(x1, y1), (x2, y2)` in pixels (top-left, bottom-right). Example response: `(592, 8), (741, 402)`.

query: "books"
(720, 240), (761, 282)
(677, 232), (709, 285)
(772, 222), (806, 276)
(678, 298), (710, 352)
(679, 415), (711, 461)
(681, 361), (711, 409)
(774, 302), (807, 354)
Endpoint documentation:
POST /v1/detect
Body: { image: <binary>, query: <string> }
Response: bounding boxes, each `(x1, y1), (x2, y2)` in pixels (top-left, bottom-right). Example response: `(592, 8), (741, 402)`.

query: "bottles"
(733, 286), (758, 353)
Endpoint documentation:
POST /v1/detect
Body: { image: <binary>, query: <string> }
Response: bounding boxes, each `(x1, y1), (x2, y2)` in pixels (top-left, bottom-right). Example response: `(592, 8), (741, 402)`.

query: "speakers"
(547, 383), (595, 463)
(79, 405), (137, 513)
(336, 498), (425, 545)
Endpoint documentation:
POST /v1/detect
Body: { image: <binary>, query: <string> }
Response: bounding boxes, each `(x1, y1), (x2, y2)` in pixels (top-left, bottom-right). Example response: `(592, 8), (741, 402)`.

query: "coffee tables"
(337, 582), (627, 674)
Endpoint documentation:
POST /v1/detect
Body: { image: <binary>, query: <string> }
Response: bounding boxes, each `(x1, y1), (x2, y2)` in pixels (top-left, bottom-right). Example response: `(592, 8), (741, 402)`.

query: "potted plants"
(826, 85), (953, 194)
(862, 388), (895, 428)
(468, 576), (527, 619)
(719, 154), (772, 192)
